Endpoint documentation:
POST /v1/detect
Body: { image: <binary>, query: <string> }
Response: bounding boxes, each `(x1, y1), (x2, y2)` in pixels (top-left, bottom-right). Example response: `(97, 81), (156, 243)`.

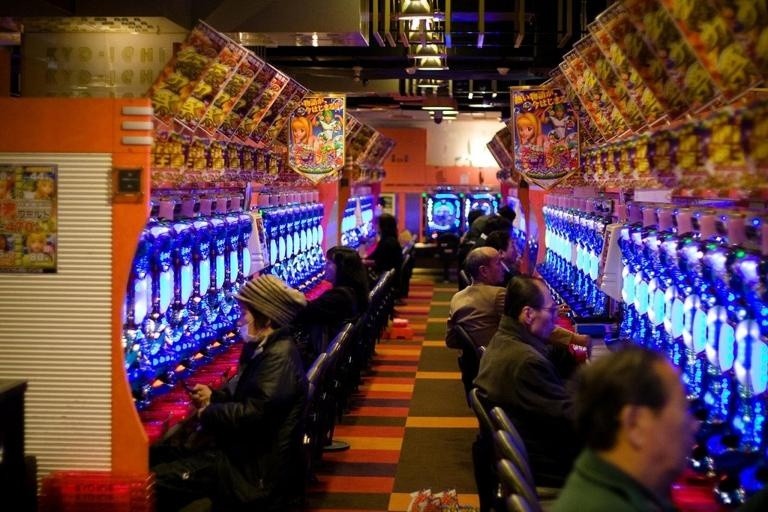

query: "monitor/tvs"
(259, 201), (331, 295)
(427, 198), (462, 240)
(617, 225), (768, 459)
(505, 196), (527, 259)
(358, 194), (376, 242)
(464, 198), (499, 232)
(533, 202), (612, 322)
(338, 198), (366, 250)
(118, 207), (253, 411)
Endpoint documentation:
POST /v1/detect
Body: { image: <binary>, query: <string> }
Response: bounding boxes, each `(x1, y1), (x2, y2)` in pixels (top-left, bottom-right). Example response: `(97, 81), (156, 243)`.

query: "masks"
(237, 319), (271, 344)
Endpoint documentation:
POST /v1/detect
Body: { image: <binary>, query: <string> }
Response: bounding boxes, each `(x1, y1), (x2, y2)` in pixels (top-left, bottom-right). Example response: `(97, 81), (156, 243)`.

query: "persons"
(292, 110), (340, 148)
(516, 105), (578, 147)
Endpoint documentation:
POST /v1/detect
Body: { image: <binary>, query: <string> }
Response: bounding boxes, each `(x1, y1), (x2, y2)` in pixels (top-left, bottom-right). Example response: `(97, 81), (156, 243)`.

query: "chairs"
(468, 387), (562, 512)
(306, 238), (417, 453)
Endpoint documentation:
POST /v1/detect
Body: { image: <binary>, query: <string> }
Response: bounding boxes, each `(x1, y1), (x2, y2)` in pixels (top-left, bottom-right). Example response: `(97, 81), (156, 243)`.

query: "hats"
(233, 275), (307, 327)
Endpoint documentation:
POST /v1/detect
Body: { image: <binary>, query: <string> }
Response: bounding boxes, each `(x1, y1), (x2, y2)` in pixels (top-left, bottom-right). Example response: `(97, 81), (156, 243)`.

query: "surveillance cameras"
(434, 116), (442, 124)
